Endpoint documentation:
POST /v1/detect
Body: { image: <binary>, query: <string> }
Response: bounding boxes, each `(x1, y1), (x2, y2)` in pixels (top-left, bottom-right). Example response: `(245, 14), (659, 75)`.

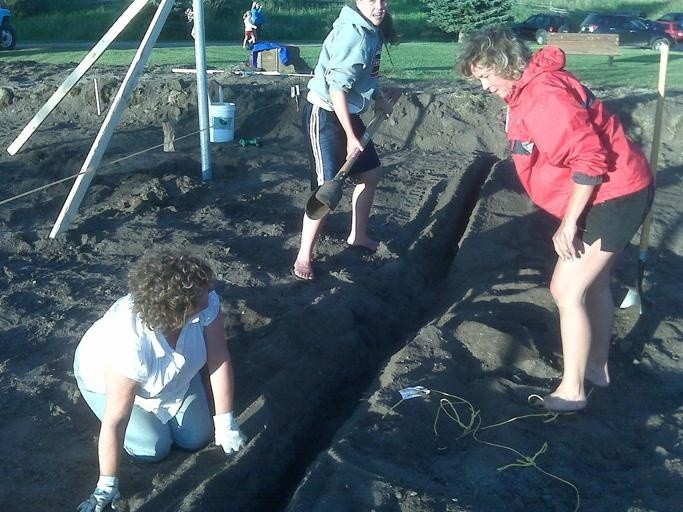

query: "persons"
(293, 0), (394, 281)
(74, 250), (249, 512)
(456, 25), (655, 413)
(243, 2), (263, 49)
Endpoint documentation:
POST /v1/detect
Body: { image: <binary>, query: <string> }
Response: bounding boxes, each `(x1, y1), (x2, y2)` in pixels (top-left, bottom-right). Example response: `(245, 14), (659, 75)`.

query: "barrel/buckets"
(210, 102), (237, 144)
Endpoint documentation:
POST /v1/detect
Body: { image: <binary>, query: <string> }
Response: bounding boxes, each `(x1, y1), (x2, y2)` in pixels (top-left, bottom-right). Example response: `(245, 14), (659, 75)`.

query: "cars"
(579, 12), (676, 51)
(0, 7), (16, 49)
(656, 13), (683, 42)
(511, 13), (572, 45)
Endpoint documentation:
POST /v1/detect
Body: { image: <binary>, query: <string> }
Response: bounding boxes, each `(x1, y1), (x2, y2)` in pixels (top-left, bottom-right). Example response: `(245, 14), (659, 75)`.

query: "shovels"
(618, 42), (669, 332)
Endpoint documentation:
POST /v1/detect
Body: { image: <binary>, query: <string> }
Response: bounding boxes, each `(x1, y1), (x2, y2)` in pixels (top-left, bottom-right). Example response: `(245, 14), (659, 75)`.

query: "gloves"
(213, 410), (247, 454)
(76, 476), (122, 512)
